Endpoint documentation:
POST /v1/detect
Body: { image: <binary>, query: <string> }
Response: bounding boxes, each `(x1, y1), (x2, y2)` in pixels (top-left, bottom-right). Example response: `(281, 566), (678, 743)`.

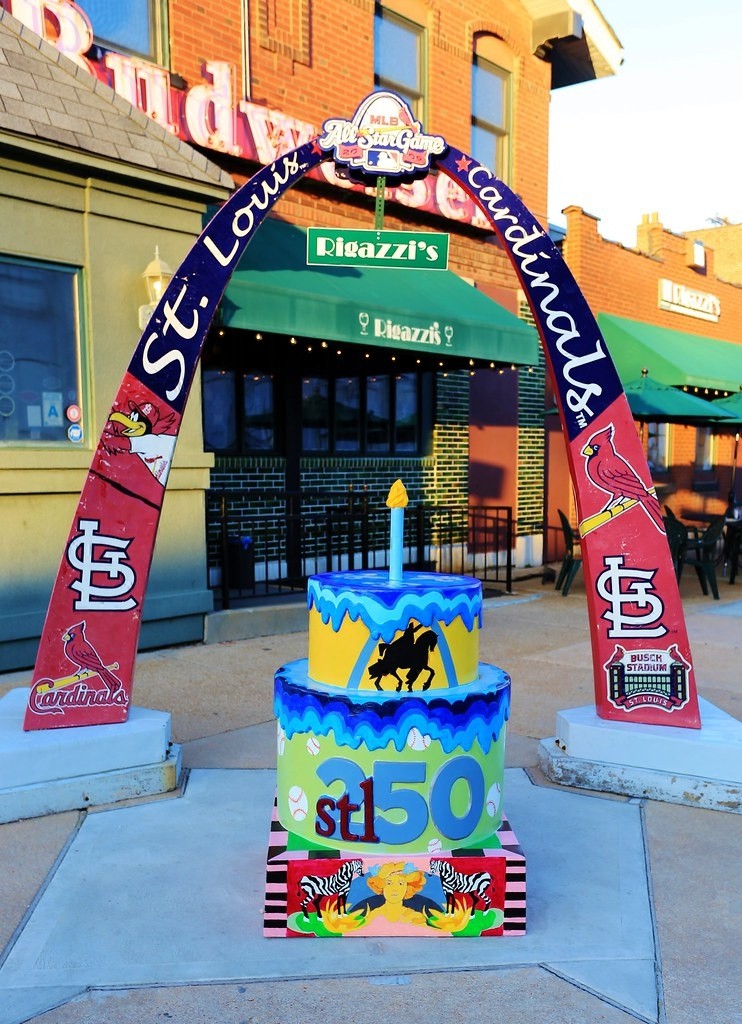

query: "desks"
(678, 512), (742, 567)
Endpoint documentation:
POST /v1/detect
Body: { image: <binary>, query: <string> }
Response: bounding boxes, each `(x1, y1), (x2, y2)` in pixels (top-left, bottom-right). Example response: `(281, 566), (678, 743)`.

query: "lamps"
(685, 239), (705, 268)
(140, 244), (175, 329)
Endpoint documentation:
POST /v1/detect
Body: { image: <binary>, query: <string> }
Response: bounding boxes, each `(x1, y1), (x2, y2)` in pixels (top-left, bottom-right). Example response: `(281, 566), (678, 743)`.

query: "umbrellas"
(537, 367), (742, 578)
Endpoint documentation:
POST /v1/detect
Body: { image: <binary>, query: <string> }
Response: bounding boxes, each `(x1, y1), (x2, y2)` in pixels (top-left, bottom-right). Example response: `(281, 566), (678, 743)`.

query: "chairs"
(555, 508), (583, 597)
(662, 486), (742, 600)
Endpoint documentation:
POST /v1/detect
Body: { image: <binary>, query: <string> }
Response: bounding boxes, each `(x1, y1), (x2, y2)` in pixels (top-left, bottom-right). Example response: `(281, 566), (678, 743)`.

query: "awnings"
(594, 312), (742, 397)
(202, 205), (539, 367)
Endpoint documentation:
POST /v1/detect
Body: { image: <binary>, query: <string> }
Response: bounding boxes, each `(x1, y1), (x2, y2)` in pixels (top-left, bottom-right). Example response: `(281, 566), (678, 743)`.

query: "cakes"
(272, 567), (511, 856)
